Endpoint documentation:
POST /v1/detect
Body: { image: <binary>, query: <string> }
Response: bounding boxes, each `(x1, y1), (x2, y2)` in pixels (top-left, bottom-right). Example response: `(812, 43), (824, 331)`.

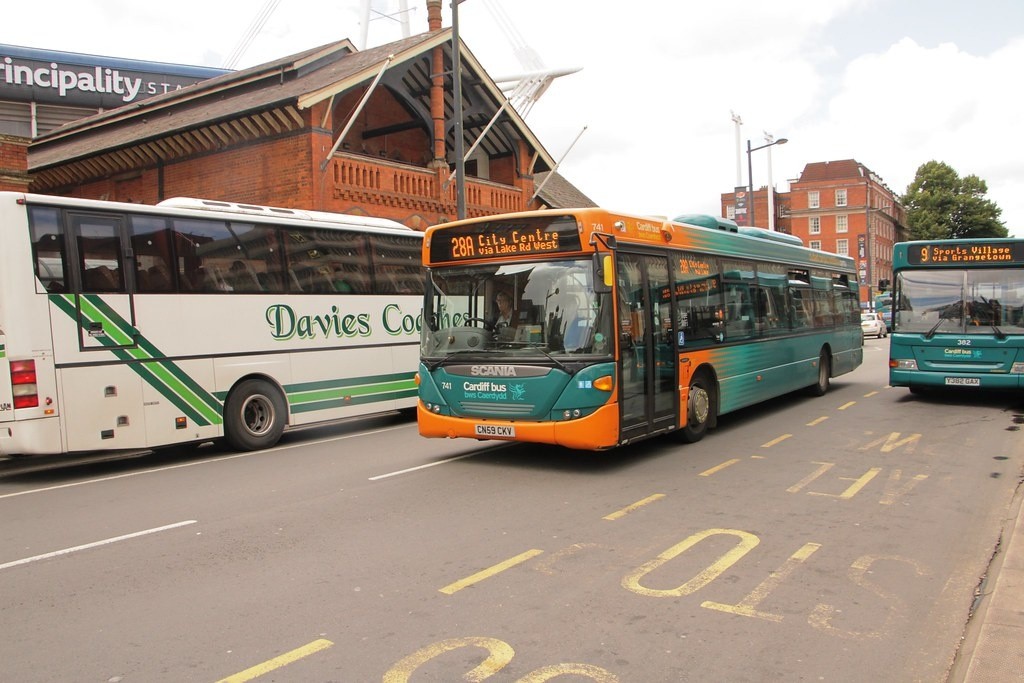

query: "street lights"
(747, 138), (789, 227)
(866, 205), (891, 313)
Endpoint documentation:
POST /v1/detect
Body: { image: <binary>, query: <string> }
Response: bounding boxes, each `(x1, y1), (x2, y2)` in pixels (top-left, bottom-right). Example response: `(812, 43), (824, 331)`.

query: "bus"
(0, 192), (484, 461)
(889, 238), (1024, 396)
(416, 207), (864, 451)
(654, 269), (860, 340)
(875, 292), (899, 329)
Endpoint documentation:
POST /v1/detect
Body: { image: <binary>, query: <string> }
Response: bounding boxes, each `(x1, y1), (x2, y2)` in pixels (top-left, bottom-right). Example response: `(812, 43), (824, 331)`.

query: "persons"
(486, 288), (523, 332)
(207, 261), (417, 293)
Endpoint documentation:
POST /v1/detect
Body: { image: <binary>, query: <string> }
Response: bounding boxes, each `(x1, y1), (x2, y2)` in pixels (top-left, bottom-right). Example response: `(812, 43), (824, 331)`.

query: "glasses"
(496, 299), (507, 303)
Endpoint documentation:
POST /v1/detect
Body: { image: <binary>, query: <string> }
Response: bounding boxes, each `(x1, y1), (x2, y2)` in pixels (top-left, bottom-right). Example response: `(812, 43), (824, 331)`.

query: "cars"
(860, 313), (888, 338)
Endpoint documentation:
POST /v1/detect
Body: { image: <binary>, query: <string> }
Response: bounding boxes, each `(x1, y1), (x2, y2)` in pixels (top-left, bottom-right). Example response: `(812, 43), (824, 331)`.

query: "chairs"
(683, 301), (846, 338)
(510, 299), (537, 329)
(38, 261), (426, 295)
